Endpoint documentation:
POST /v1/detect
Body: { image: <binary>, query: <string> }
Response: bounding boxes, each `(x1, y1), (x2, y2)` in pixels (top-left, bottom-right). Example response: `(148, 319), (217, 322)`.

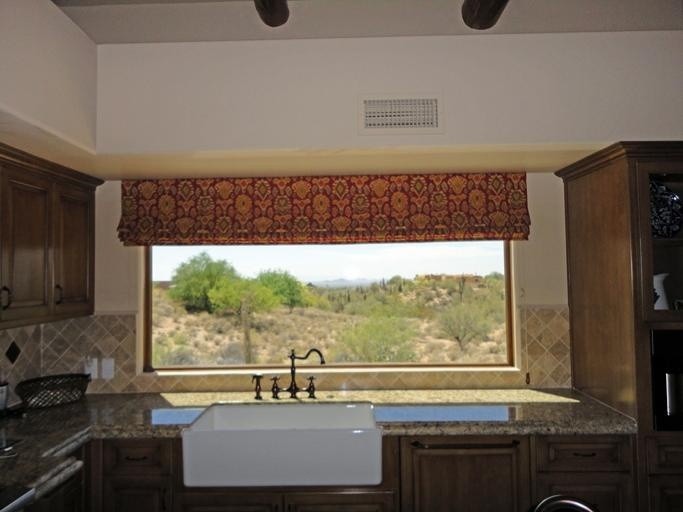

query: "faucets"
(284, 347), (324, 398)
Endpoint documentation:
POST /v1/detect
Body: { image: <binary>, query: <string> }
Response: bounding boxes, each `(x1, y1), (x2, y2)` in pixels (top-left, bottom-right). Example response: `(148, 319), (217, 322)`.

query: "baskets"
(14, 372), (92, 409)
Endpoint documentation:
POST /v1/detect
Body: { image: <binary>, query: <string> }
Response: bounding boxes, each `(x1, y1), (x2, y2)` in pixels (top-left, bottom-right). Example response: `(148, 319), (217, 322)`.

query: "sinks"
(179, 403), (385, 488)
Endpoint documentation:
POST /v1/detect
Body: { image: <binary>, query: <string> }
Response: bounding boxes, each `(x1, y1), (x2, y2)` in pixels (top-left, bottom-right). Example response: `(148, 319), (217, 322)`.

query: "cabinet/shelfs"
(0, 156), (95, 331)
(565, 157), (682, 511)
(531, 437), (636, 511)
(89, 438), (182, 511)
(400, 434), (529, 509)
